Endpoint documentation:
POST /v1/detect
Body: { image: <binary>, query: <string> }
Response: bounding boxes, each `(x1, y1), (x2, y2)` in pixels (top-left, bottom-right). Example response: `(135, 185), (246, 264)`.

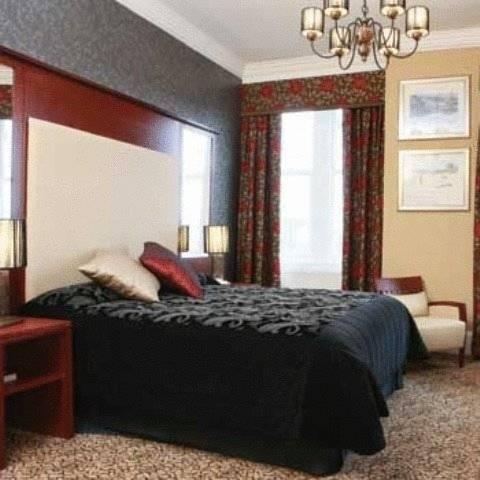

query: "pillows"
(81, 248), (162, 302)
(390, 290), (429, 317)
(139, 241), (204, 298)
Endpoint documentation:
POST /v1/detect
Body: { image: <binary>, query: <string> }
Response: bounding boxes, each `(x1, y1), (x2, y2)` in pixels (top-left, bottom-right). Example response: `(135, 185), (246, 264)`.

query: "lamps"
(202, 224), (231, 284)
(299, 1), (429, 72)
(178, 224), (189, 253)
(1, 219), (28, 328)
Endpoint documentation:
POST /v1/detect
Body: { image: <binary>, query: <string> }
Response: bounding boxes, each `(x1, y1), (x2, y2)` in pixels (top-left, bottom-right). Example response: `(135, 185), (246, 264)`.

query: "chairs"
(372, 275), (468, 368)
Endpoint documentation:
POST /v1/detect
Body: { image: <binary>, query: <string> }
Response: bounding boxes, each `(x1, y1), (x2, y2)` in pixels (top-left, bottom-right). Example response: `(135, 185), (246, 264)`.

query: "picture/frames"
(397, 73), (471, 141)
(395, 146), (471, 212)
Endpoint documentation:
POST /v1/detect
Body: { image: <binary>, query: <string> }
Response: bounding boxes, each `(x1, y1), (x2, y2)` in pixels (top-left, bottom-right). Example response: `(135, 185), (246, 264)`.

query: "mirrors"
(177, 127), (213, 281)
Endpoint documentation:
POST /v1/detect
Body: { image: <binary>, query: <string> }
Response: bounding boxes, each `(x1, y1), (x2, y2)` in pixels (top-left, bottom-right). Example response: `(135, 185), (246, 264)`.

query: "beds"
(14, 52), (428, 480)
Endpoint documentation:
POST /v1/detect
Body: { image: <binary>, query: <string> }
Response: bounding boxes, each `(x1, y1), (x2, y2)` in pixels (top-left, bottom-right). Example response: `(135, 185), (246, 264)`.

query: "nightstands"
(1, 318), (75, 469)
